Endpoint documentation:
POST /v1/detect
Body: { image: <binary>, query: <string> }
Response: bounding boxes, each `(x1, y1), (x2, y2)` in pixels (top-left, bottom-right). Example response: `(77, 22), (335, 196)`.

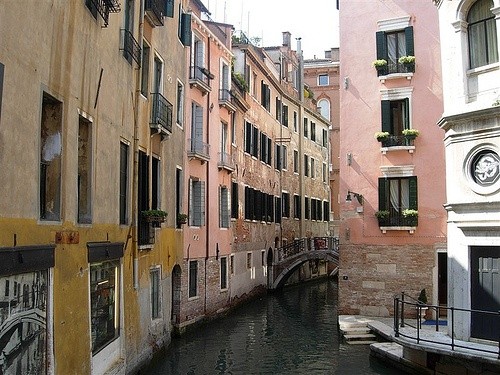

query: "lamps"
(345, 190), (362, 206)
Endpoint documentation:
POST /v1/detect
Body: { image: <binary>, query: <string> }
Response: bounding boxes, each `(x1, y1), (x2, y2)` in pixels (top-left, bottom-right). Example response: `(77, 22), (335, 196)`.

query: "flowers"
(397, 56), (416, 64)
(401, 128), (419, 136)
(401, 209), (418, 219)
(374, 210), (390, 218)
(376, 131), (390, 139)
(370, 59), (387, 66)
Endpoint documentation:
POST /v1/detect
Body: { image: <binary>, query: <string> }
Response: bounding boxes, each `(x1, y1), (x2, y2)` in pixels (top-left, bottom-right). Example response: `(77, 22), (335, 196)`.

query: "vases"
(404, 62), (414, 67)
(378, 66), (386, 70)
(403, 215), (415, 218)
(377, 137), (389, 142)
(406, 135), (417, 138)
(379, 216), (386, 220)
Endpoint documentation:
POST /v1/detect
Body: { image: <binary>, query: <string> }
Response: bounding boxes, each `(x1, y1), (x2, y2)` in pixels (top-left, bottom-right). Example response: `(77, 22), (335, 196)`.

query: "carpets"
(423, 320), (447, 326)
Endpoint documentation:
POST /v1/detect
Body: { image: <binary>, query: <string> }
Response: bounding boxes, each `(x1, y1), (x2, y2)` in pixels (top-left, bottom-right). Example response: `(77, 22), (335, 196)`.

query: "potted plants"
(415, 288), (428, 323)
(140, 209), (168, 223)
(177, 213), (188, 224)
